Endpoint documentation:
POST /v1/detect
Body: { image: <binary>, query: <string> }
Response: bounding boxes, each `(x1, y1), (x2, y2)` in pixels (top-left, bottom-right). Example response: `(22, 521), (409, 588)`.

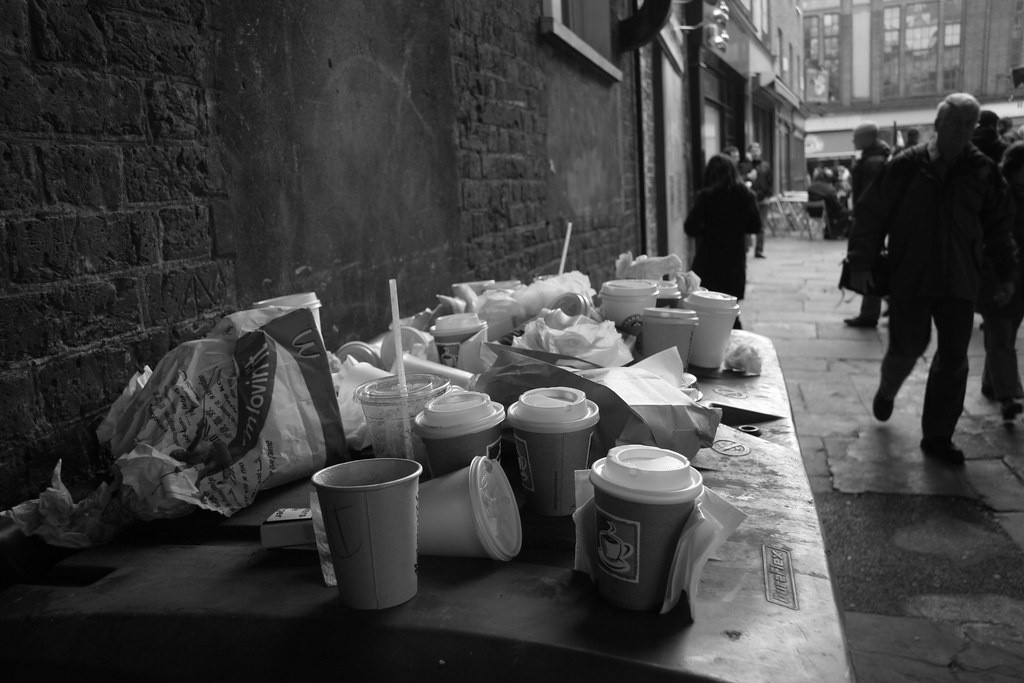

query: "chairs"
(798, 200), (831, 241)
(764, 190), (812, 241)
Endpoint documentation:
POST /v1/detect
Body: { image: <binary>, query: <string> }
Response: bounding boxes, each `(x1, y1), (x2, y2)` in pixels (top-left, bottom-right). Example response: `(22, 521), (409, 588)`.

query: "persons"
(807, 92), (1024, 469)
(683, 142), (772, 331)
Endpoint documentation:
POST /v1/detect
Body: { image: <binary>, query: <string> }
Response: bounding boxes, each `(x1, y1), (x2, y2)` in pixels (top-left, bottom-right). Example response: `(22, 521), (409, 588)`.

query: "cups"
(588, 442), (704, 614)
(507, 385), (600, 518)
(335, 312), (489, 372)
(311, 457), (424, 612)
(352, 371), (451, 461)
(546, 277), (742, 375)
(417, 454), (523, 562)
(411, 390), (507, 477)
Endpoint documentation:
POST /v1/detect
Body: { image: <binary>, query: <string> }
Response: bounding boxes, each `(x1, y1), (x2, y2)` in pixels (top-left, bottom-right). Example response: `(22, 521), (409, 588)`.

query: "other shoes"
(873, 383), (894, 421)
(1001, 402), (1022, 420)
(844, 313), (878, 328)
(920, 438), (963, 466)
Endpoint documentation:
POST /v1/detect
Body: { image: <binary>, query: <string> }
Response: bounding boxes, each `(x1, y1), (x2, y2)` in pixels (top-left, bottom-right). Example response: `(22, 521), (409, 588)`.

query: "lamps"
(669, 0), (730, 29)
(704, 24), (731, 53)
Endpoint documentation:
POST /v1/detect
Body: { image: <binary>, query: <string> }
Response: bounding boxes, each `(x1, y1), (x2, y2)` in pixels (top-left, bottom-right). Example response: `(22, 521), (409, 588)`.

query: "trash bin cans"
(0, 327), (853, 683)
